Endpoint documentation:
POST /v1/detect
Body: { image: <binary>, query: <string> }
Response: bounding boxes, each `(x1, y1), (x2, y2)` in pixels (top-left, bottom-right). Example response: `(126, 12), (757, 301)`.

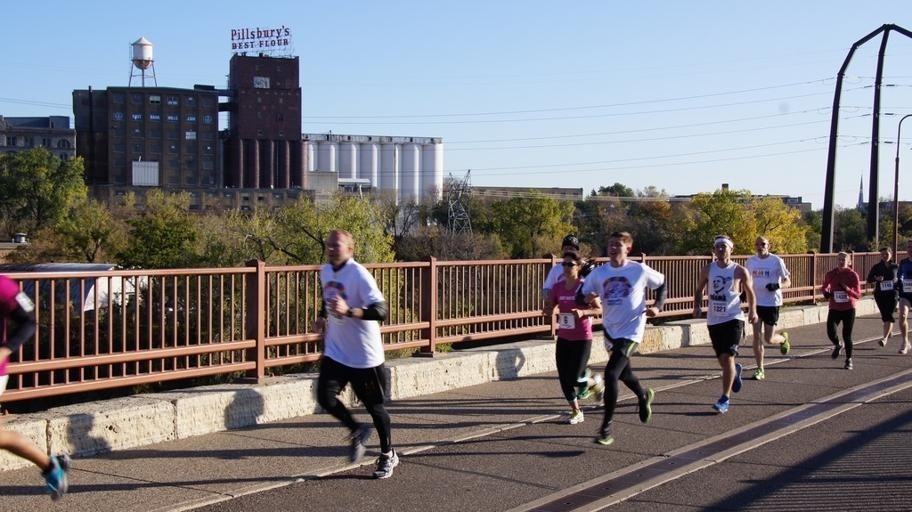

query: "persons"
(821, 251), (862, 370)
(574, 230), (668, 445)
(866, 246), (900, 348)
(745, 236), (791, 379)
(541, 234), (603, 399)
(540, 249), (607, 424)
(0, 273), (72, 501)
(315, 229), (399, 480)
(691, 234), (759, 413)
(897, 239), (912, 355)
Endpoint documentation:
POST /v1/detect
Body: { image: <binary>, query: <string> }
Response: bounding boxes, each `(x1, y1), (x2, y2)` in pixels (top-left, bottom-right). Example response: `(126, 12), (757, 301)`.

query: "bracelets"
(345, 306), (355, 316)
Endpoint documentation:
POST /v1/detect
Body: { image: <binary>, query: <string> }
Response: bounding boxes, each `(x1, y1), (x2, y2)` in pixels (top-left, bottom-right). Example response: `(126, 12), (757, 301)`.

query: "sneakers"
(832, 341), (844, 359)
(899, 341), (912, 355)
(878, 331), (892, 347)
(711, 395), (730, 414)
(844, 357), (853, 370)
(752, 368), (765, 379)
(349, 422), (372, 463)
(568, 408), (585, 425)
(732, 363), (741, 393)
(373, 448), (400, 480)
(638, 387), (655, 425)
(577, 373), (604, 402)
(781, 332), (790, 355)
(39, 452), (72, 504)
(597, 434), (615, 446)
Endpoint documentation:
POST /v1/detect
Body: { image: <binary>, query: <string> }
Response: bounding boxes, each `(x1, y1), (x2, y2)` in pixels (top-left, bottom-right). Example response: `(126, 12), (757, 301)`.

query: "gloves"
(738, 290), (748, 304)
(765, 282), (779, 292)
